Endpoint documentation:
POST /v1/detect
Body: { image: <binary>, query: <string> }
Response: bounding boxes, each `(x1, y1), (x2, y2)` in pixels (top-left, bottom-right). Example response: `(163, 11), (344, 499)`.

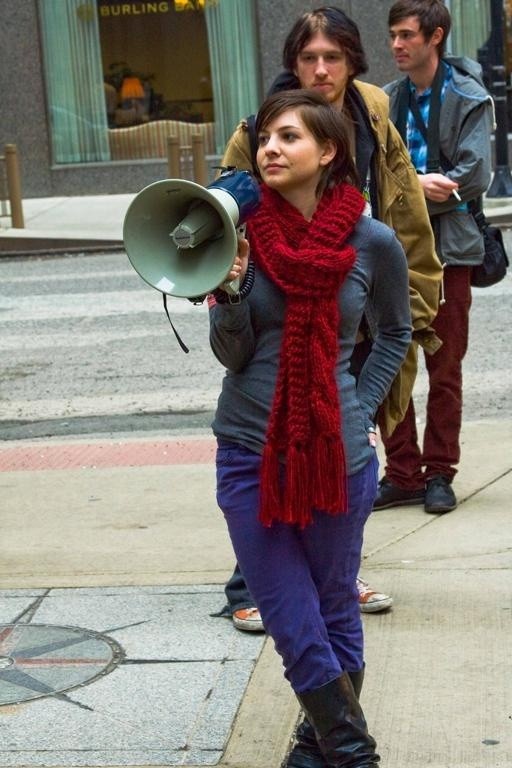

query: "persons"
(372, 0), (495, 513)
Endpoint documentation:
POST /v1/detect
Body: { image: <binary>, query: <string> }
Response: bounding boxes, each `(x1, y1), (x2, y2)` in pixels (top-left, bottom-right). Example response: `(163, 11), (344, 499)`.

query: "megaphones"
(122, 168), (261, 299)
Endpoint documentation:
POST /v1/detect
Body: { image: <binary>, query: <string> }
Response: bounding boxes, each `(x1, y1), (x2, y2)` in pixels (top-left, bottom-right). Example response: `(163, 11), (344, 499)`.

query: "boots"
(280, 662), (381, 768)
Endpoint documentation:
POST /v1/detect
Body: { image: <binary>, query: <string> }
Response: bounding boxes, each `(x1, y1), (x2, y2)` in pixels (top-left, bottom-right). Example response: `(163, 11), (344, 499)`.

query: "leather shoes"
(370, 472), (457, 514)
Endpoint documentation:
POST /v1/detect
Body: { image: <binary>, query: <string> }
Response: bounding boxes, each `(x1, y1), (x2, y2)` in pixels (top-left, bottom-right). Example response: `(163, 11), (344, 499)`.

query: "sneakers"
(357, 576), (394, 614)
(232, 607), (267, 632)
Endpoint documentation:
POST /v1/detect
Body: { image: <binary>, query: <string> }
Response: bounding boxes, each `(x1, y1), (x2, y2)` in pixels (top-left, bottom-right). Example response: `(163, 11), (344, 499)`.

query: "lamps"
(122, 76), (145, 111)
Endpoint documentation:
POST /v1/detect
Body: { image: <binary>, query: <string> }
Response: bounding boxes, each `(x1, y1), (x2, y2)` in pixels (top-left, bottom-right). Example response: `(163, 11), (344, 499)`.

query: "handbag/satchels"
(470, 222), (509, 288)
